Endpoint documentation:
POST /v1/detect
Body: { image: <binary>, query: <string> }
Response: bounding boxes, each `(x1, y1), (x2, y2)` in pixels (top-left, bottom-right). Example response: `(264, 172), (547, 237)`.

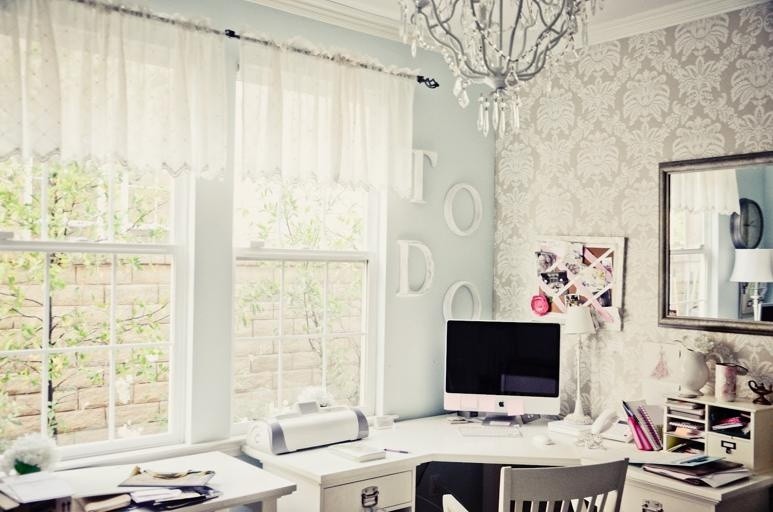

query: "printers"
(244, 402), (370, 456)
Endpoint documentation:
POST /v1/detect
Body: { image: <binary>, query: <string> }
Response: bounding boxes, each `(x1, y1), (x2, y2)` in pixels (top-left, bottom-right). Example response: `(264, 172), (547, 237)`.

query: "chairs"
(443, 459), (629, 512)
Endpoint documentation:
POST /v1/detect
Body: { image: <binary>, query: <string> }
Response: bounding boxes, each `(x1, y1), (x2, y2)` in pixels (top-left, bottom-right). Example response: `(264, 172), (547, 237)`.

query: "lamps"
(397, 0), (605, 140)
(728, 248), (772, 324)
(562, 306), (597, 426)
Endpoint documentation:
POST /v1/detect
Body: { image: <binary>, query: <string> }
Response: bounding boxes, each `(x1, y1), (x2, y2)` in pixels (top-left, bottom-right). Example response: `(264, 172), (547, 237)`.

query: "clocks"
(729, 196), (763, 249)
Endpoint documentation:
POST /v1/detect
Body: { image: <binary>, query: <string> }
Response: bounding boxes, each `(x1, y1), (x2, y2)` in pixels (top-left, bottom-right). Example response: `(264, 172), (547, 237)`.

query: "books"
(621, 399), (665, 451)
(665, 398), (705, 440)
(72, 465), (222, 512)
(711, 411), (753, 438)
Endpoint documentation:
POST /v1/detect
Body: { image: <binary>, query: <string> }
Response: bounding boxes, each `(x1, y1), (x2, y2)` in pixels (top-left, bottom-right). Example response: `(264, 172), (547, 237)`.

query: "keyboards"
(460, 426), (522, 438)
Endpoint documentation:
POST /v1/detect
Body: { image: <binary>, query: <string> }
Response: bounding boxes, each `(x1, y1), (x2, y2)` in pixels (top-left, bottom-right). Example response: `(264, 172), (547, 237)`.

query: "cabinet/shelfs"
(662, 395), (773, 475)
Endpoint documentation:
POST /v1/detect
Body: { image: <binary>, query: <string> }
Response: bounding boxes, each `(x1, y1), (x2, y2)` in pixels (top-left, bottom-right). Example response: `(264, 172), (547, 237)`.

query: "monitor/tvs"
(442, 319), (562, 424)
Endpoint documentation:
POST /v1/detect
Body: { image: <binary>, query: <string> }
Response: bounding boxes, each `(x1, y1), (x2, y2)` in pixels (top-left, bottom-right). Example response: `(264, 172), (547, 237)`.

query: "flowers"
(531, 295), (554, 317)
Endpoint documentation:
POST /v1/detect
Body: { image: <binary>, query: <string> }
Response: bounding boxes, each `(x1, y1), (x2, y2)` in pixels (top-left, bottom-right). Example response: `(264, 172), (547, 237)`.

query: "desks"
(65, 449), (297, 511)
(241, 408), (773, 511)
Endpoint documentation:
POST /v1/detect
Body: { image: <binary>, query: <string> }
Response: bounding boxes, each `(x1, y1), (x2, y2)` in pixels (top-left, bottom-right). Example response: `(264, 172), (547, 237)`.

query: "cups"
(715, 363), (736, 401)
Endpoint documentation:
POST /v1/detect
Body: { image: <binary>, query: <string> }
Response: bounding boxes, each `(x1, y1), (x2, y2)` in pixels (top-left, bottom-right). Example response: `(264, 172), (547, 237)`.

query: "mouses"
(537, 432), (552, 445)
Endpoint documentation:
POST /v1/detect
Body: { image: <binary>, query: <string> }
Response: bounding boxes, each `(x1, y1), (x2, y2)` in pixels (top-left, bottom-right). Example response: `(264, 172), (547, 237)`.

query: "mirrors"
(658, 150), (772, 337)
